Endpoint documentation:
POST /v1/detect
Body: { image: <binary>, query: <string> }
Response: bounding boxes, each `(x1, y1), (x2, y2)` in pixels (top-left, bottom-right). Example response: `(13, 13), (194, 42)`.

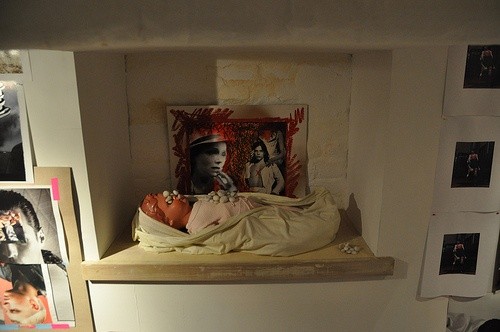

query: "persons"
(244, 141), (284, 195)
(139, 191), (340, 257)
(453, 239), (466, 264)
(186, 136), (237, 195)
(0, 190), (70, 324)
(467, 149), (480, 177)
(255, 128), (286, 167)
(479, 45), (495, 75)
